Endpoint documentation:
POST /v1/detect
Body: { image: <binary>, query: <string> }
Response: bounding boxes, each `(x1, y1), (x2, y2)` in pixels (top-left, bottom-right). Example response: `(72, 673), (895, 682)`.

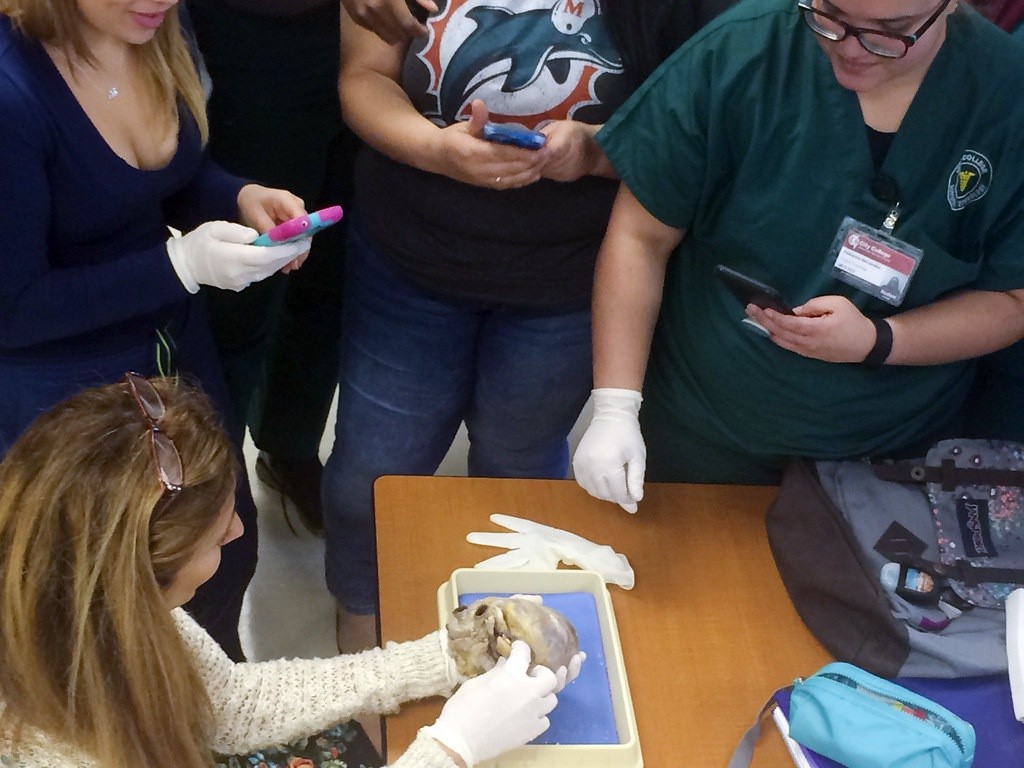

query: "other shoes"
(336, 607), (379, 655)
(256, 454), (324, 538)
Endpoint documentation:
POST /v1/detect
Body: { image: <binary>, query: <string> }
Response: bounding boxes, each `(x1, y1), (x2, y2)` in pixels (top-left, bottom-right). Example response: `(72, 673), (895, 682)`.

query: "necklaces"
(49, 41), (125, 100)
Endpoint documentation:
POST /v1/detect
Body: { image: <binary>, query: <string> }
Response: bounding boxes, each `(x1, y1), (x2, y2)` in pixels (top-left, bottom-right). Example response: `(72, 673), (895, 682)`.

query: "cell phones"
(252, 205), (344, 246)
(716, 264), (796, 319)
(483, 124), (547, 152)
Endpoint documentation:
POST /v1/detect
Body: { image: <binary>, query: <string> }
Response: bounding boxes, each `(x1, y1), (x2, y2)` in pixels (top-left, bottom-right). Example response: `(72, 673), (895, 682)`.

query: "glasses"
(797, 0), (950, 60)
(118, 371), (183, 500)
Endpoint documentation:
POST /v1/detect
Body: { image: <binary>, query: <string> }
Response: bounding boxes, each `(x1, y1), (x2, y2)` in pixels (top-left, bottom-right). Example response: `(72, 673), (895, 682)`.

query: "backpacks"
(764, 434), (1024, 680)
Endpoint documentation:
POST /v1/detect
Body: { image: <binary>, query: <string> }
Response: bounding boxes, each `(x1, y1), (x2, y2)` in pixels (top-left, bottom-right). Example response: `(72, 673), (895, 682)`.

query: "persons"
(0, 370), (587, 768)
(0, 0), (1024, 665)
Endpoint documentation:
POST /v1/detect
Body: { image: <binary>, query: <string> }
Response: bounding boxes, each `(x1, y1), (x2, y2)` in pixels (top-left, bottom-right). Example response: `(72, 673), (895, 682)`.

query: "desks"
(372, 476), (836, 768)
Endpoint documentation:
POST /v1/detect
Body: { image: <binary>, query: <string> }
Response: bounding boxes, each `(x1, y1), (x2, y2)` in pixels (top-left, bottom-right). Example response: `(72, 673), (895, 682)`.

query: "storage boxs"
(437, 568), (644, 768)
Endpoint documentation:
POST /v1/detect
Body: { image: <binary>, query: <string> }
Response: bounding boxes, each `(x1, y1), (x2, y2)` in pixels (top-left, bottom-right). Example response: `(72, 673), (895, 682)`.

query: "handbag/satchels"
(727, 659), (1024, 768)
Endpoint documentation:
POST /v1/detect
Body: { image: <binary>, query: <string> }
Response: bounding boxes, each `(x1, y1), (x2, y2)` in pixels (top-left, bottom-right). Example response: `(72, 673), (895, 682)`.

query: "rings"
(495, 177), (500, 183)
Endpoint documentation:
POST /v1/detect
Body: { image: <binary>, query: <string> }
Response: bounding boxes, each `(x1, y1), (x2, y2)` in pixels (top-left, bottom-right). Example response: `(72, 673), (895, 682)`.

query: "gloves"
(465, 513), (635, 590)
(425, 640), (558, 768)
(438, 594), (588, 695)
(573, 388), (651, 513)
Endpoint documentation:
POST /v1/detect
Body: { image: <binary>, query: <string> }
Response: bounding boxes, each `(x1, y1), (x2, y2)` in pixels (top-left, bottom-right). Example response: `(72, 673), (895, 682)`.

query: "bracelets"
(861, 316), (893, 367)
